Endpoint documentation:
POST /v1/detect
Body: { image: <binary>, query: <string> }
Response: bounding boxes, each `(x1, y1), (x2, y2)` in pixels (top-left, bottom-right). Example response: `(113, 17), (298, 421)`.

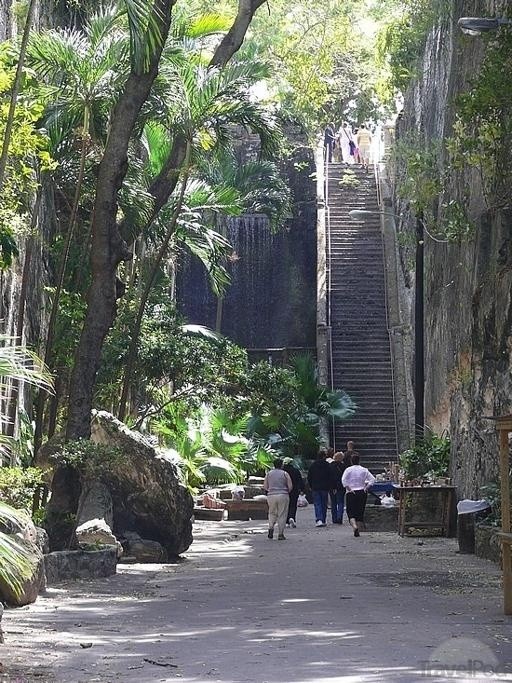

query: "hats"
(283, 456), (293, 465)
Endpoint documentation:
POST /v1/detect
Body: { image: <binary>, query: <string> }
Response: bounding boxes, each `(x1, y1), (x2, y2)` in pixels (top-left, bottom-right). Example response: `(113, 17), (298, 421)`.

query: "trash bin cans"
(457, 501), (491, 553)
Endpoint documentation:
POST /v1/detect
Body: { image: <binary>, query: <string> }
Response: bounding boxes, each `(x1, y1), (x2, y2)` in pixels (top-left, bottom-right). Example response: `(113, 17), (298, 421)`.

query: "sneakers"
(278, 533), (285, 539)
(354, 527), (359, 536)
(316, 519), (322, 526)
(268, 529), (273, 538)
(289, 517), (296, 527)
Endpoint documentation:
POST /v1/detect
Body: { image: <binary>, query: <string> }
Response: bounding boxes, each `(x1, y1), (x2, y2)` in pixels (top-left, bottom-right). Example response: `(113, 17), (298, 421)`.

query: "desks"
(392, 480), (458, 539)
(366, 480), (393, 505)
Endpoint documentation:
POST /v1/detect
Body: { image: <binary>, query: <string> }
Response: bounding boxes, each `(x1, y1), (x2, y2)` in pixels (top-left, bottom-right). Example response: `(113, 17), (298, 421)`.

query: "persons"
(329, 451), (347, 524)
(281, 456), (304, 528)
(325, 121), (372, 163)
(263, 457), (293, 540)
(342, 440), (360, 467)
(341, 454), (375, 536)
(307, 450), (331, 526)
